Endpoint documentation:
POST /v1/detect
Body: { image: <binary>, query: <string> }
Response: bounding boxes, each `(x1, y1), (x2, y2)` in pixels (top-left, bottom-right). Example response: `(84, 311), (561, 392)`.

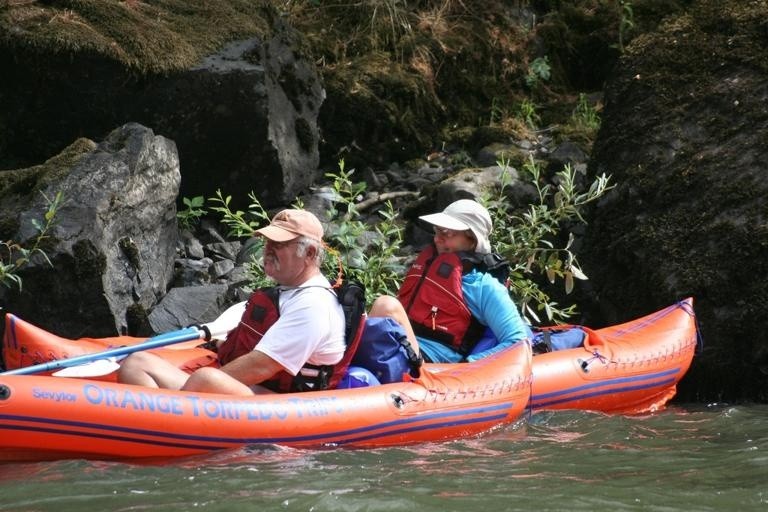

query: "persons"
(118, 209), (347, 400)
(368, 199), (527, 364)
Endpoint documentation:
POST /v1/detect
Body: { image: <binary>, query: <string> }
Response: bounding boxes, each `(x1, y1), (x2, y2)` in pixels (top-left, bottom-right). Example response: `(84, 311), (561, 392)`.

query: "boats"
(2, 296), (699, 422)
(0, 335), (533, 461)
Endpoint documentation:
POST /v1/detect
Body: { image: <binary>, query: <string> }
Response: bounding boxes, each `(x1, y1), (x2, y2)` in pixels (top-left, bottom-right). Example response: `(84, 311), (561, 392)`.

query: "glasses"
(433, 226), (455, 237)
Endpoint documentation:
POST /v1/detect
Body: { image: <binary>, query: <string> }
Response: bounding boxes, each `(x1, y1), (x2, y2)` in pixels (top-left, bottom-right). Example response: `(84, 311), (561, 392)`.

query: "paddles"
(0, 299), (249, 377)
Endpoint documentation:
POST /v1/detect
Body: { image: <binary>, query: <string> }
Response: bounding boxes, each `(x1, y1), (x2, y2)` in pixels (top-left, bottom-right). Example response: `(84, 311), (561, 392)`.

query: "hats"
(418, 198), (493, 254)
(254, 209), (324, 243)
